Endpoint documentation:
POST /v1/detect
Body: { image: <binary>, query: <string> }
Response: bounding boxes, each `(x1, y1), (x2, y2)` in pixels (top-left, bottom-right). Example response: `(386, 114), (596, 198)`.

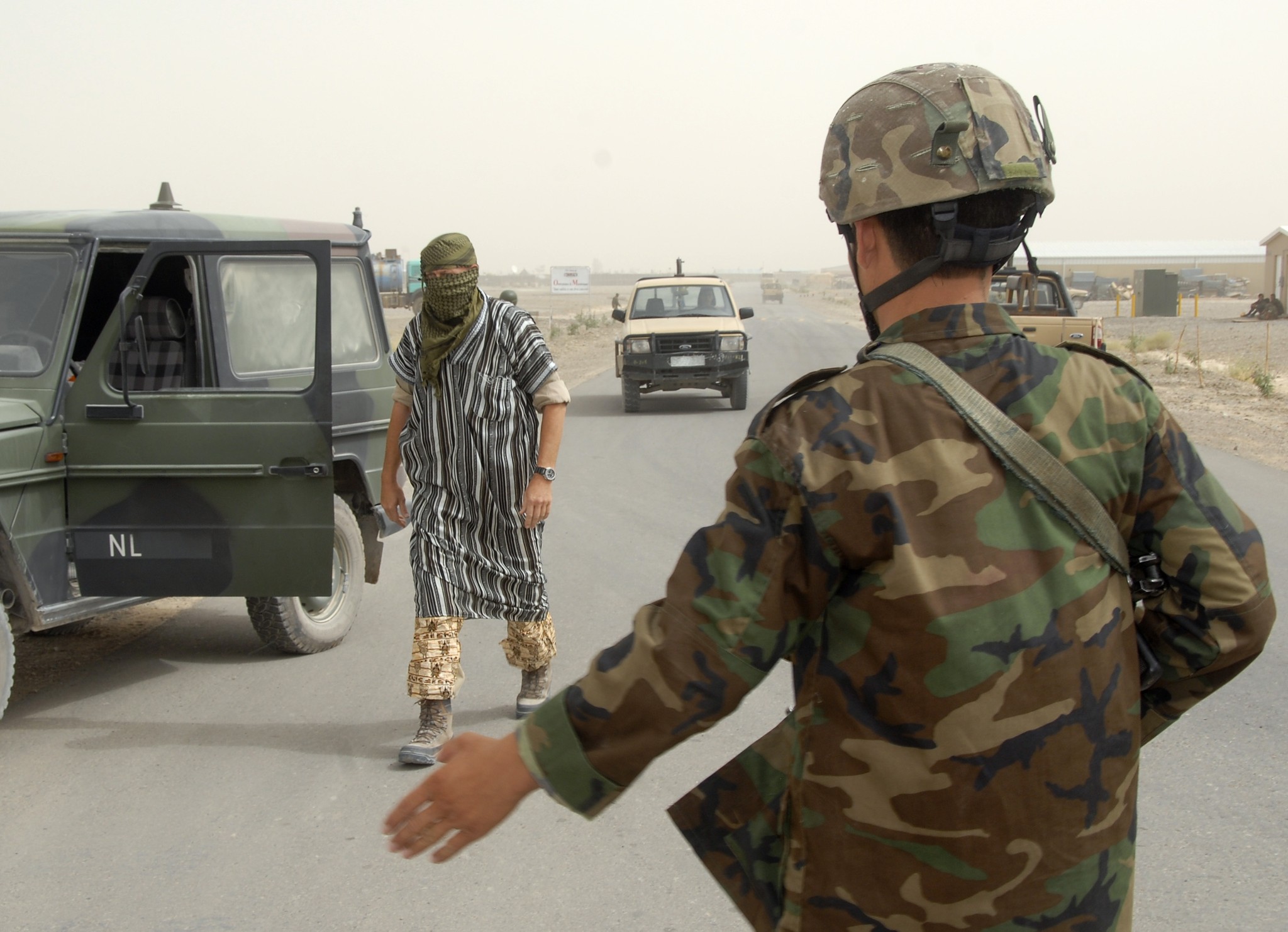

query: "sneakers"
(397, 697), (455, 765)
(515, 657), (551, 717)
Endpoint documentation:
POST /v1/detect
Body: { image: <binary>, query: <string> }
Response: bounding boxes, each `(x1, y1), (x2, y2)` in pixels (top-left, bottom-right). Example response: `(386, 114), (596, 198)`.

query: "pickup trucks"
(989, 252), (1108, 353)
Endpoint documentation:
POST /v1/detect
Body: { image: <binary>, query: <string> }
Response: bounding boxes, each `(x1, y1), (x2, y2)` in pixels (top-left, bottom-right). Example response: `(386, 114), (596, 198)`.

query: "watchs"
(534, 467), (556, 481)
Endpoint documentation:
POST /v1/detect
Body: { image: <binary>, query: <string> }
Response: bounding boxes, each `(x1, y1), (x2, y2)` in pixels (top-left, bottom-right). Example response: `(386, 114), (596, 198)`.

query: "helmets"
(819, 60), (1063, 224)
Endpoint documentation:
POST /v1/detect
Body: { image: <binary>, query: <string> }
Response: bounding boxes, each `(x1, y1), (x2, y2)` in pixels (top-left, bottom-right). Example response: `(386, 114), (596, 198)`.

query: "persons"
(1245, 294), (1284, 320)
(612, 293), (621, 310)
(698, 286), (716, 308)
(381, 233), (571, 764)
(381, 63), (1278, 932)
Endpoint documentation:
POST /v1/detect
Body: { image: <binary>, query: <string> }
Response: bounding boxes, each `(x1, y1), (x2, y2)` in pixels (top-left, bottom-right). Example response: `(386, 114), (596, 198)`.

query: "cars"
(760, 273), (779, 289)
(1066, 286), (1090, 309)
(762, 282), (784, 305)
(611, 256), (755, 413)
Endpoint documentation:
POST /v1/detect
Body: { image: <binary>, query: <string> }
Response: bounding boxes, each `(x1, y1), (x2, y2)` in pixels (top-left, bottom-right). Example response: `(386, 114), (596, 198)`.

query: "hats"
(419, 233), (477, 273)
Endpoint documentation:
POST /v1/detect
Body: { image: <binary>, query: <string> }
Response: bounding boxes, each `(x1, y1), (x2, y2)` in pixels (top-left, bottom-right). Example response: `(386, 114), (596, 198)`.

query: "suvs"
(0, 179), (415, 733)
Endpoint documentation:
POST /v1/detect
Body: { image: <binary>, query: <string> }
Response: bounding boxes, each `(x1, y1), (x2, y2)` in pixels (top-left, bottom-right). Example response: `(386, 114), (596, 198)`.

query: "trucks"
(370, 248), (427, 315)
